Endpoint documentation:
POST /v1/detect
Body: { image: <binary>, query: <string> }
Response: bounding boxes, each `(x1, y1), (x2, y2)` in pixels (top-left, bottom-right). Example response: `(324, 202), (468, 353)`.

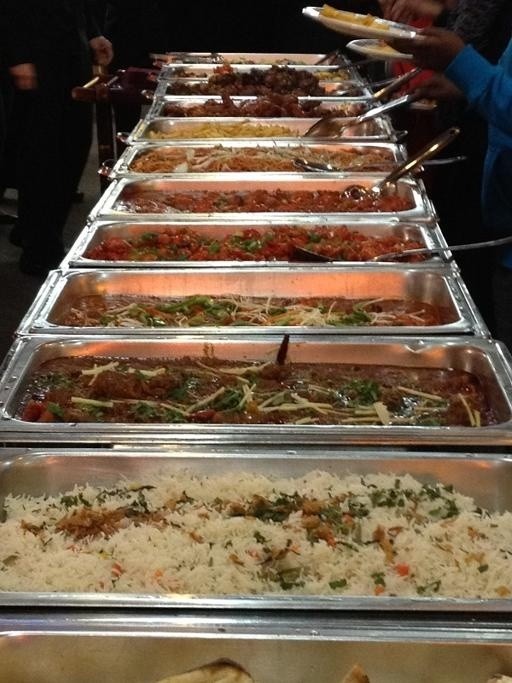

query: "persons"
(368, 0), (512, 306)
(0, 2), (115, 299)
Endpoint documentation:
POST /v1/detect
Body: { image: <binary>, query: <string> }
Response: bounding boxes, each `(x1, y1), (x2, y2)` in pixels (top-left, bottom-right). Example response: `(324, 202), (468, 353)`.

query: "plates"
(301, 5), (416, 38)
(345, 39), (414, 61)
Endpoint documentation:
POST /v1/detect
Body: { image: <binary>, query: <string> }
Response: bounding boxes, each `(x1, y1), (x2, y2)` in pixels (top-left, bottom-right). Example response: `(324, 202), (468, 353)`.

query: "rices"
(1, 467), (512, 600)
